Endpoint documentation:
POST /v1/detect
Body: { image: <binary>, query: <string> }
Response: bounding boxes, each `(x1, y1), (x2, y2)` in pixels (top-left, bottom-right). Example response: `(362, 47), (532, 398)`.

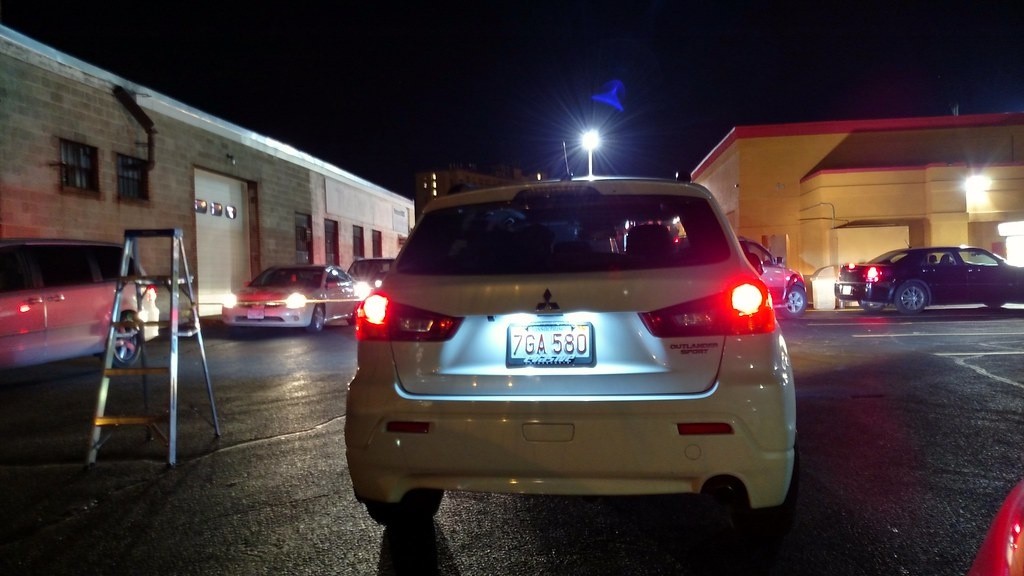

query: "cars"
(346, 258), (399, 297)
(835, 246), (1023, 316)
(734, 236), (809, 315)
(223, 265), (371, 333)
(343, 176), (801, 526)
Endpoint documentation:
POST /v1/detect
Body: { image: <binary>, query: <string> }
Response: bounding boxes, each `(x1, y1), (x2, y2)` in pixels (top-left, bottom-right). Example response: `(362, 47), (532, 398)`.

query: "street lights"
(583, 129), (601, 177)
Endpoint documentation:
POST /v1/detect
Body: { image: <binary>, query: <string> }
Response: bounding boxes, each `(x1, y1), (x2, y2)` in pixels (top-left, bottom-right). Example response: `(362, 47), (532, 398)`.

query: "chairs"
(929, 255), (936, 263)
(939, 254), (953, 264)
(626, 224), (675, 258)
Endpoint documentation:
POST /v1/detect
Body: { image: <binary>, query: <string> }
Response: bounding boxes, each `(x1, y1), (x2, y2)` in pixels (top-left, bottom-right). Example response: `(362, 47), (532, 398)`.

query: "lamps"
(227, 154), (236, 165)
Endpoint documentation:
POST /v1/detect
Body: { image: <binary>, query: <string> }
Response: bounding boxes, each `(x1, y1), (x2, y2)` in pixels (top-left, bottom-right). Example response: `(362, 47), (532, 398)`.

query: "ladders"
(86, 225), (220, 465)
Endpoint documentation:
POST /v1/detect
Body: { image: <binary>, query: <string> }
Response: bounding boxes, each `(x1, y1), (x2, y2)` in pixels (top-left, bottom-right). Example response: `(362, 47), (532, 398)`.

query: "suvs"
(0, 238), (162, 369)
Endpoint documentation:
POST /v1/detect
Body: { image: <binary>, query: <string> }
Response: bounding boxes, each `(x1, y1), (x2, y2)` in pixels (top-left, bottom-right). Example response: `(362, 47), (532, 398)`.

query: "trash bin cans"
(810, 265), (836, 310)
